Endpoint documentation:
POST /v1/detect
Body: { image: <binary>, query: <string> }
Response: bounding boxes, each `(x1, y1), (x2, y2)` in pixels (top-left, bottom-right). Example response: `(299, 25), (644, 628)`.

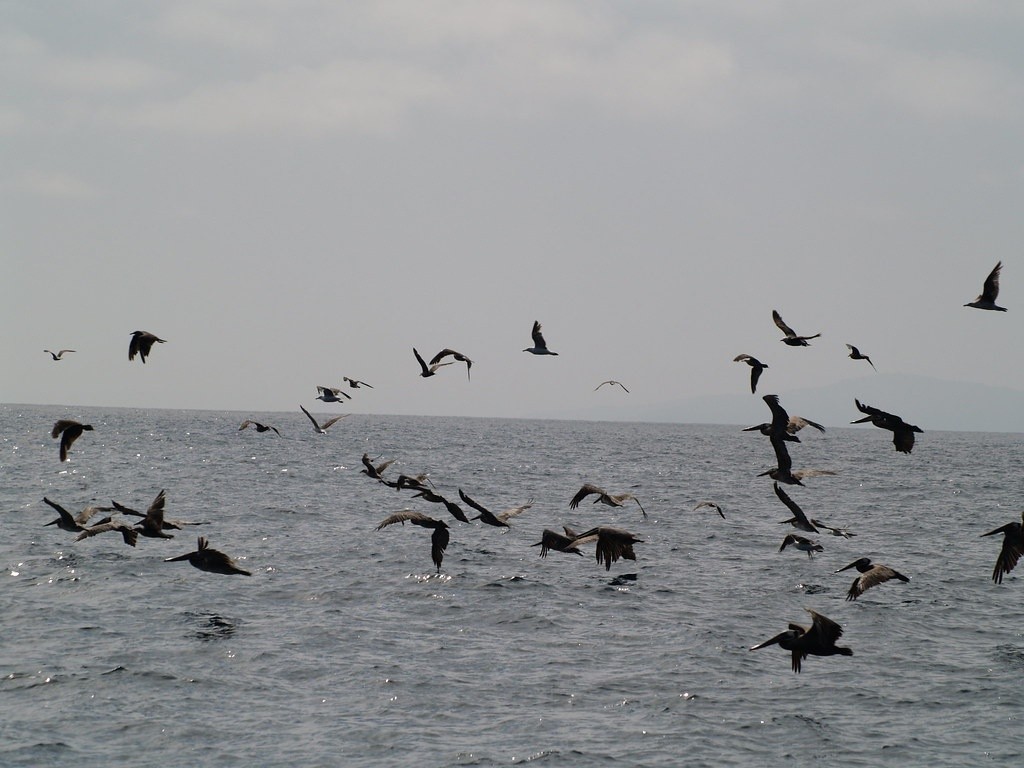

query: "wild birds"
(53, 419), (94, 463)
(128, 331), (167, 364)
(692, 500), (726, 521)
(569, 484), (649, 519)
(834, 557), (911, 602)
(316, 377), (374, 404)
(412, 346), (455, 378)
(529, 524), (584, 558)
(732, 258), (1008, 560)
(163, 535), (251, 577)
(239, 419), (281, 438)
(595, 381), (630, 393)
(430, 348), (472, 381)
(44, 349), (76, 361)
(979, 512), (1024, 584)
(360, 451), (539, 572)
(576, 526), (645, 571)
(750, 605), (853, 676)
(298, 405), (352, 435)
(522, 320), (559, 357)
(40, 495), (212, 549)
